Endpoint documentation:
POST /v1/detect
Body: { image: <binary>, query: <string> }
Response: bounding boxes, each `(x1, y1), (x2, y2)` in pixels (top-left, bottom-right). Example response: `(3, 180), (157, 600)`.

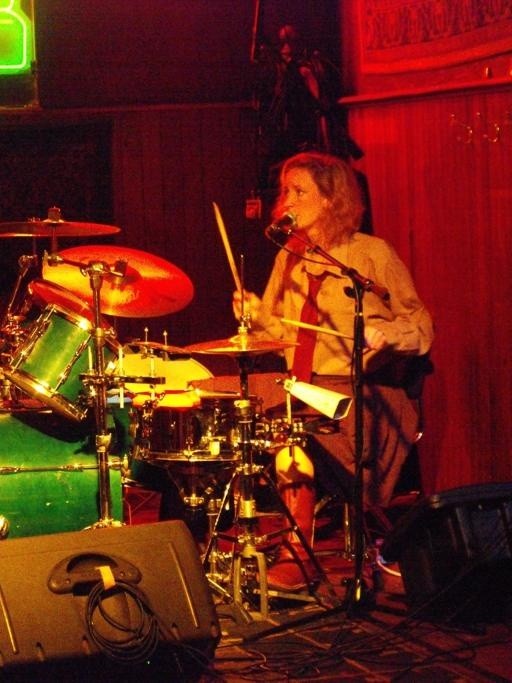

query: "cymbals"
(1, 220), (121, 241)
(185, 333), (299, 356)
(28, 277), (111, 332)
(42, 245), (196, 319)
(123, 342), (191, 361)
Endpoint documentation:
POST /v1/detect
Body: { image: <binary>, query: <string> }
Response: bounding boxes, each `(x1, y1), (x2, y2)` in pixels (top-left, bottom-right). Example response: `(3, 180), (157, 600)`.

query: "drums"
(137, 390), (256, 461)
(109, 347), (214, 476)
(0, 399), (125, 539)
(1, 303), (124, 422)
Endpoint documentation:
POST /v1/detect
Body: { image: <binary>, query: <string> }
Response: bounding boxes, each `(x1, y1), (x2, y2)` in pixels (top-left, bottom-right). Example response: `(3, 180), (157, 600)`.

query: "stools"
(319, 428), (423, 586)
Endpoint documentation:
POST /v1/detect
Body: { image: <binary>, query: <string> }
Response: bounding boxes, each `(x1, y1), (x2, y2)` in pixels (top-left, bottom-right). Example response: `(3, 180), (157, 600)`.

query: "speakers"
(0, 517), (223, 682)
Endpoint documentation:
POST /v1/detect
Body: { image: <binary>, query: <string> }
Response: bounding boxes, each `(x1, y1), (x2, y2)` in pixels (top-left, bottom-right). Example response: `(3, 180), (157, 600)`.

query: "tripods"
(241, 290), (512, 683)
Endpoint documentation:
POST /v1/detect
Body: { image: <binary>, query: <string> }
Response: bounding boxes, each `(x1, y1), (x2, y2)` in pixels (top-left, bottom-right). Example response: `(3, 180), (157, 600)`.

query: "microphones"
(267, 211), (297, 231)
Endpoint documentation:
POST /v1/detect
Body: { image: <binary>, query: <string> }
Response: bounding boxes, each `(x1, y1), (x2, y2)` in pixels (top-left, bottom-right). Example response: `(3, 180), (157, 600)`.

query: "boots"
(257, 473), (315, 592)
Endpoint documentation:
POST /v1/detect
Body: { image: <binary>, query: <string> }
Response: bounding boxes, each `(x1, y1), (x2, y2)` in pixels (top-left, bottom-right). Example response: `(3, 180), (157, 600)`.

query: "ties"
(291, 266), (328, 403)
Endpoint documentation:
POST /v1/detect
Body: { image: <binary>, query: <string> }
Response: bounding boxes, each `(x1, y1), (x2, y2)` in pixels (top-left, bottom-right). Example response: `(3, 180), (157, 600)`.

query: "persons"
(233, 153), (433, 592)
